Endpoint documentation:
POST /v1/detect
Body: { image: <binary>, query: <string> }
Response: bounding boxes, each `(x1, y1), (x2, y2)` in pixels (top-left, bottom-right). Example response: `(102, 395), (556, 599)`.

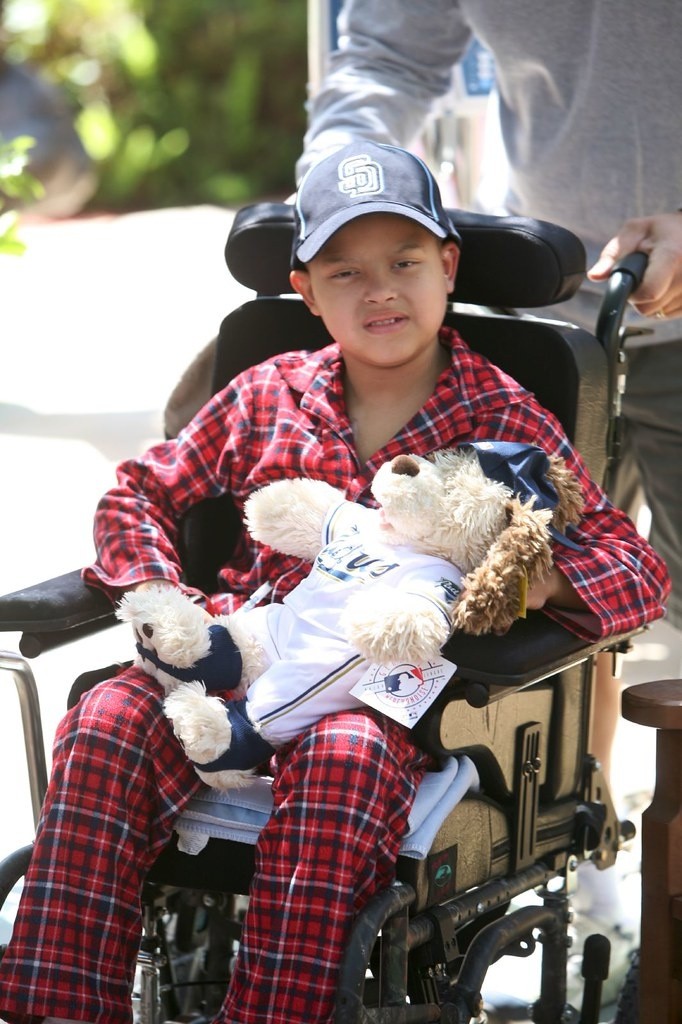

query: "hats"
(291, 139), (462, 270)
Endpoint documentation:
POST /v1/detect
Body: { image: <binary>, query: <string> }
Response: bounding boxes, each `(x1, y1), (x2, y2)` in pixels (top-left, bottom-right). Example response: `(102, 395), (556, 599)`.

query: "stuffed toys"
(119, 441), (582, 788)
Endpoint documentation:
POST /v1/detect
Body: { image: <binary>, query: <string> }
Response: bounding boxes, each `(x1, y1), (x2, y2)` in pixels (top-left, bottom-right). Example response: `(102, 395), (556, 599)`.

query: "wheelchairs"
(0, 204), (654, 1024)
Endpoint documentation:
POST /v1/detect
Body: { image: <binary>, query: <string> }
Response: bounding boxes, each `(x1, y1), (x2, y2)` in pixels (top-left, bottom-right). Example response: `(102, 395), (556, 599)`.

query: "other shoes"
(560, 909), (635, 1009)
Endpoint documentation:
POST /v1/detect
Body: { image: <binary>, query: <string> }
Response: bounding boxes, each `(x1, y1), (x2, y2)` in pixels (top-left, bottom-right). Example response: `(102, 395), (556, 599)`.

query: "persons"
(297, 0), (682, 1012)
(0, 144), (669, 1024)
